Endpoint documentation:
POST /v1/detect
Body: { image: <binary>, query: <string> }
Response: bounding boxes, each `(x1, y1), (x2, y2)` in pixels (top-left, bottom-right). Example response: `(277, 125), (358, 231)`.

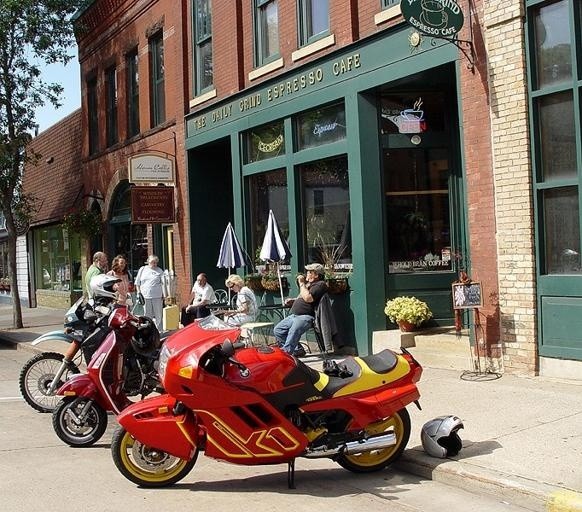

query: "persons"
(106, 254), (136, 313)
(134, 255), (168, 338)
(221, 273), (259, 325)
(273, 262), (329, 357)
(85, 251), (109, 307)
(181, 273), (219, 329)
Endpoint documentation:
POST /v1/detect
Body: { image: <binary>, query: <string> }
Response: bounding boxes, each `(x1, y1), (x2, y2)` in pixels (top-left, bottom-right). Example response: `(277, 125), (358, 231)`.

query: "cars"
(121, 235), (148, 262)
(273, 201), (353, 263)
(42, 254), (70, 288)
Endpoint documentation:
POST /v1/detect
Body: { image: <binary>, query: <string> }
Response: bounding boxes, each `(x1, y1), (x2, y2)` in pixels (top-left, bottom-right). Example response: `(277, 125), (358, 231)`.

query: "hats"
(305, 263), (326, 276)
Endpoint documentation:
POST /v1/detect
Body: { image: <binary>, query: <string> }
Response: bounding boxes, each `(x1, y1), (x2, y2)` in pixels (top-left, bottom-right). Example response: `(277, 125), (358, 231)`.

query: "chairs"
(214, 289), (227, 303)
(308, 293), (330, 360)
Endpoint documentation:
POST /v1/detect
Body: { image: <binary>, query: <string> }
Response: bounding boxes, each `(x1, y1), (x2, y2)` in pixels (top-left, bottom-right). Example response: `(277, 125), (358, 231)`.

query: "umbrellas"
(216, 221), (249, 305)
(259, 209), (293, 318)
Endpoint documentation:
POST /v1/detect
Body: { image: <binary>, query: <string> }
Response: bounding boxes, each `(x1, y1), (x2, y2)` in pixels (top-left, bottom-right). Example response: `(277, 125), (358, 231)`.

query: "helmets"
(421, 416), (464, 459)
(90, 274), (122, 301)
(131, 315), (160, 354)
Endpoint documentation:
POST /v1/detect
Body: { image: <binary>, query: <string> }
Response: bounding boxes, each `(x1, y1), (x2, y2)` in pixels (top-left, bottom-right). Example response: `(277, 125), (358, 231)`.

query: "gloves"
(322, 359), (352, 378)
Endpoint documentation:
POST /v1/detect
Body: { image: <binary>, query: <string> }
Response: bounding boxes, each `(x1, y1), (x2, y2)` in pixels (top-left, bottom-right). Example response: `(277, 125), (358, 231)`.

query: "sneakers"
(294, 347), (306, 357)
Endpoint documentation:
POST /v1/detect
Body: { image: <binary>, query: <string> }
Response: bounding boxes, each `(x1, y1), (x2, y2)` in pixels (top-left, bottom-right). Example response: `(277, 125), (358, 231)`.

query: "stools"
(240, 322), (273, 348)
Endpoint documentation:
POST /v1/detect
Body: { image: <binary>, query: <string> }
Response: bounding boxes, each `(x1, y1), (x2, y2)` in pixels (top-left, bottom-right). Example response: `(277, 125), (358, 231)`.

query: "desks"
(205, 305), (236, 323)
(258, 305), (312, 354)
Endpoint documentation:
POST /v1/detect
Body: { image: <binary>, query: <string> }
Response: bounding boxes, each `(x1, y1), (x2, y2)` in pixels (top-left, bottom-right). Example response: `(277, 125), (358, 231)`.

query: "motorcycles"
(20, 272), (183, 413)
(52, 294), (246, 446)
(111, 307), (423, 489)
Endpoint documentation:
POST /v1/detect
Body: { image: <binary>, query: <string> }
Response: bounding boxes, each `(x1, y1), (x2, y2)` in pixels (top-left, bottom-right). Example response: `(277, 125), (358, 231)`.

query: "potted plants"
(384, 296), (434, 332)
